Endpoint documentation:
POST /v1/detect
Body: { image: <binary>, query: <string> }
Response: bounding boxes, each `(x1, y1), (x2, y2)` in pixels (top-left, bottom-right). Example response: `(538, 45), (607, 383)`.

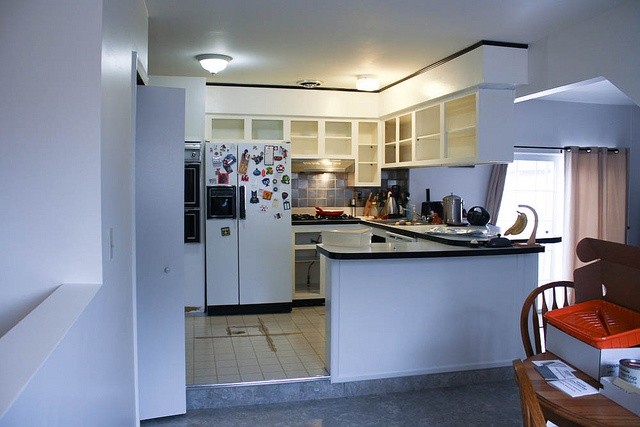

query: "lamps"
(193, 54), (233, 76)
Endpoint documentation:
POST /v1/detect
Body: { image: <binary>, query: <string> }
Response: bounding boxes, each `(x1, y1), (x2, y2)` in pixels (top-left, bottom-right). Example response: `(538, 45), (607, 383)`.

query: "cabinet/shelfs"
(209, 118), (285, 141)
(288, 119), (353, 159)
(353, 121), (382, 187)
(381, 88), (515, 168)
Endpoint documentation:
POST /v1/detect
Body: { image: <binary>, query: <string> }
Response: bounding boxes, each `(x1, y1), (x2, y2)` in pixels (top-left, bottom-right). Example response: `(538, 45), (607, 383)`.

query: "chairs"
(520, 278), (579, 356)
(508, 358), (548, 427)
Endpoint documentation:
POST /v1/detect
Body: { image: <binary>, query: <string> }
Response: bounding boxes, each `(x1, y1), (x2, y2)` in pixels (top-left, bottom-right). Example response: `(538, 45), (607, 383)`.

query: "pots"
(315, 206), (344, 216)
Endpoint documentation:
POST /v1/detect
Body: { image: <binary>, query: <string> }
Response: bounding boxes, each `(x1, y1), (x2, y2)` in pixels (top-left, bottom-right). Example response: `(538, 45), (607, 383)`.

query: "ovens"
(183, 141), (201, 243)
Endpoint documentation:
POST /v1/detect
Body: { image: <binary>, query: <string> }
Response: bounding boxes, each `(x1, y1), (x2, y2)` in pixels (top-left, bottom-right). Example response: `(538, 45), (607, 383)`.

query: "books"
(548, 378), (600, 397)
(532, 360), (576, 372)
(533, 366), (576, 381)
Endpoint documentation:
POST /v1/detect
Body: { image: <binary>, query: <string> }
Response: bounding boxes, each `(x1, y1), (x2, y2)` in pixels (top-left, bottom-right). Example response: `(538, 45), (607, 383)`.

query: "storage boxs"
(320, 228), (371, 248)
(542, 298), (640, 382)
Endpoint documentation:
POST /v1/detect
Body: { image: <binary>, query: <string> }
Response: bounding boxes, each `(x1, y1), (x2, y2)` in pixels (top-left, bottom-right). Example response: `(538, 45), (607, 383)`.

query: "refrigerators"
(204, 139), (294, 316)
